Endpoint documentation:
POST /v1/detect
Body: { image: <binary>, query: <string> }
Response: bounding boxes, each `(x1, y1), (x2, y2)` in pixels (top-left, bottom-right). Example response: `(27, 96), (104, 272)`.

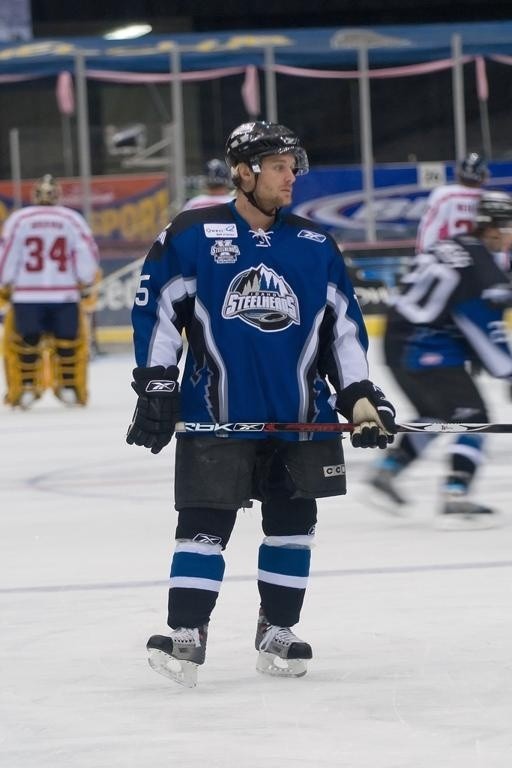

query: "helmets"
(476, 192), (512, 230)
(31, 173), (63, 207)
(206, 158), (234, 188)
(457, 150), (490, 184)
(223, 118), (304, 166)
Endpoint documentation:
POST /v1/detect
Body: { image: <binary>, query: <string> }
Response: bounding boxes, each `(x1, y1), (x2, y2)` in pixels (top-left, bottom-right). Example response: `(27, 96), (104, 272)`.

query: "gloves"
(329, 378), (396, 450)
(123, 363), (183, 456)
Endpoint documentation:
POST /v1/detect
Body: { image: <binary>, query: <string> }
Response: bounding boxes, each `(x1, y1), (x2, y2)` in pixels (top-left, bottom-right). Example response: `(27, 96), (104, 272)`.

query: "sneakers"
(372, 466), (404, 504)
(146, 621), (206, 666)
(441, 496), (493, 515)
(14, 388), (81, 411)
(253, 608), (314, 659)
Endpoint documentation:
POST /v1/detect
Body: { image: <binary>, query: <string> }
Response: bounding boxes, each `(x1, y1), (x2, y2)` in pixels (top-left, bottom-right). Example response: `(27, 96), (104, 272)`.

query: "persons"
(411, 151), (493, 255)
(124, 121), (399, 666)
(0, 172), (104, 411)
(181, 158), (238, 213)
(366, 188), (512, 514)
